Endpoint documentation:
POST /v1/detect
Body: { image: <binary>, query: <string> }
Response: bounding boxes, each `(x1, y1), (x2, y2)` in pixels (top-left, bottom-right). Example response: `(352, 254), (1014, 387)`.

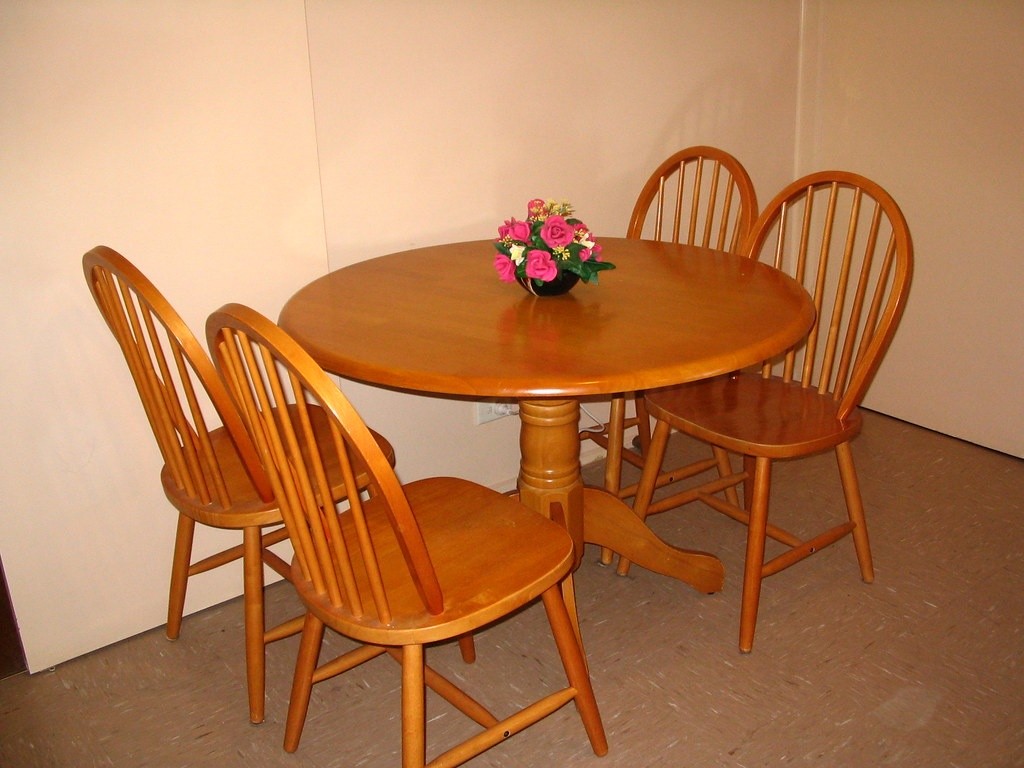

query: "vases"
(514, 269), (581, 296)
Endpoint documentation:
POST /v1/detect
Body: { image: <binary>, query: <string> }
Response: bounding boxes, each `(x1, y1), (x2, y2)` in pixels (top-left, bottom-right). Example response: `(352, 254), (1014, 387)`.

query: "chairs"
(81, 144), (916, 768)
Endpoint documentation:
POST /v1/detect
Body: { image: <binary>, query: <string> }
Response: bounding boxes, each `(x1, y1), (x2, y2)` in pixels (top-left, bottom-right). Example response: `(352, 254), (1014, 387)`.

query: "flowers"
(494, 197), (616, 287)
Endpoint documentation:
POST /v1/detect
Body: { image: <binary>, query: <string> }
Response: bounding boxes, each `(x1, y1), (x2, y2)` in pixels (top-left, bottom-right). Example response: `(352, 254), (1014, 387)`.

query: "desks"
(275, 236), (819, 653)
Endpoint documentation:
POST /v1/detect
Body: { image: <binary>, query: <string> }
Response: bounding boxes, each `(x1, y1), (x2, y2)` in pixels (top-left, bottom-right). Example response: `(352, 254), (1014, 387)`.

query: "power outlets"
(474, 401), (513, 426)
